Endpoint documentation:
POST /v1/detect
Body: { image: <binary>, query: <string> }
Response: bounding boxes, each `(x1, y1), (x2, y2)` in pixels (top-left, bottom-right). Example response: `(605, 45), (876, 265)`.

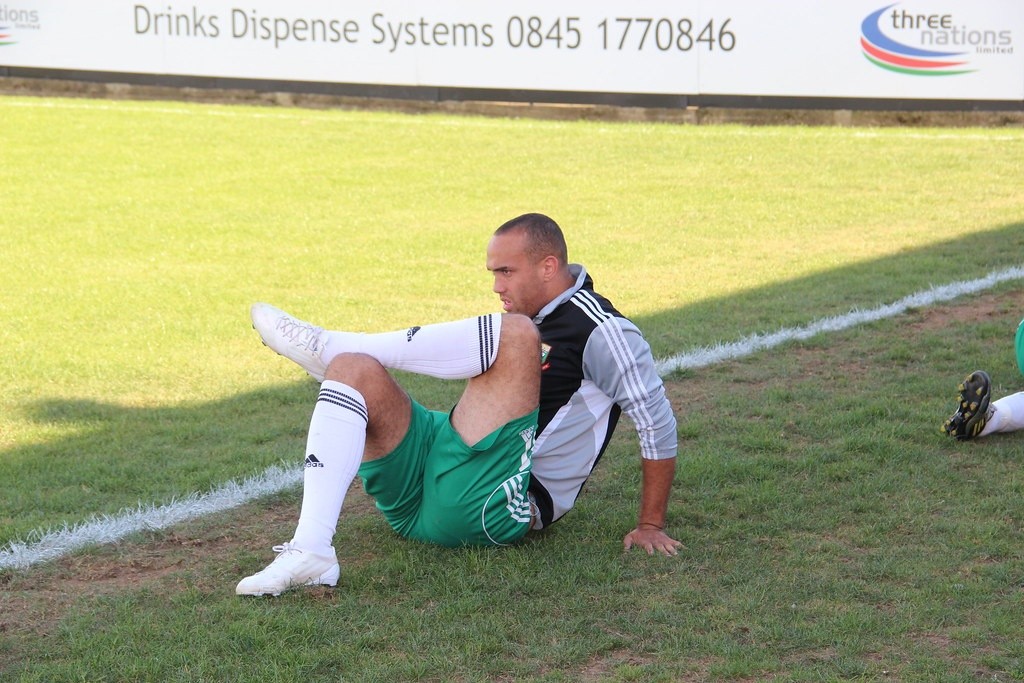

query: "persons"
(234, 211), (689, 597)
(940, 318), (1024, 442)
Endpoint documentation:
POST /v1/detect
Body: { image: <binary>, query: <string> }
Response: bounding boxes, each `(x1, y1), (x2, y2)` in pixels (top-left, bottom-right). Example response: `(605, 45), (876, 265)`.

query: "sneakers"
(249, 302), (328, 385)
(941, 369), (992, 442)
(235, 540), (340, 596)
